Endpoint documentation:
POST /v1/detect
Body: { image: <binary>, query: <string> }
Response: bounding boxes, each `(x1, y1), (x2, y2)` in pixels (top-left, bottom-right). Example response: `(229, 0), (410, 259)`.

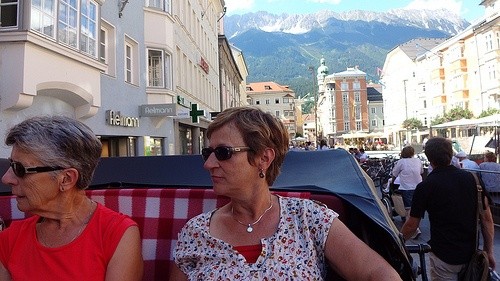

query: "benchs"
(0, 189), (343, 281)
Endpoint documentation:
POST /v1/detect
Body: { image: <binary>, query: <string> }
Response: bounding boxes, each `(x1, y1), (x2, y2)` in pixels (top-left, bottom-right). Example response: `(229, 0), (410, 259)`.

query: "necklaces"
(40, 199), (93, 247)
(231, 195), (273, 232)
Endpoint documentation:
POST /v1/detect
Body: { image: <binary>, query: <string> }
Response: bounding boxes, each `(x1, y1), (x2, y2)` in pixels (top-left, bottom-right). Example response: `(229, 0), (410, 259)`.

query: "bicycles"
(361, 152), (429, 220)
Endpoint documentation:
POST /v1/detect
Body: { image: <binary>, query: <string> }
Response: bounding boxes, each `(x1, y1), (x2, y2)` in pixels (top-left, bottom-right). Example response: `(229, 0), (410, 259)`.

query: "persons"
(386, 137), (500, 281)
(0, 117), (144, 281)
(172, 106), (403, 281)
(289, 138), (383, 168)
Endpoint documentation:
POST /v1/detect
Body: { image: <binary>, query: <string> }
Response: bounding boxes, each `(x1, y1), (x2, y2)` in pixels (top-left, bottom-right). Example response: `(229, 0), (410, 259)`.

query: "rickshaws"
(0, 148), (432, 281)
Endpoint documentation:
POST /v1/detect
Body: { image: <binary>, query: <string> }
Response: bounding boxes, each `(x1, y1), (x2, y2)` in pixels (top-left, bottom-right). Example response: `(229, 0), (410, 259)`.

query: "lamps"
(217, 7), (227, 22)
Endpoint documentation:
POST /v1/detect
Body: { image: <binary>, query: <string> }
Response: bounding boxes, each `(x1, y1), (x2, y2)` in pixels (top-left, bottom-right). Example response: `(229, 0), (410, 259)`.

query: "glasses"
(8, 157), (66, 178)
(202, 145), (252, 162)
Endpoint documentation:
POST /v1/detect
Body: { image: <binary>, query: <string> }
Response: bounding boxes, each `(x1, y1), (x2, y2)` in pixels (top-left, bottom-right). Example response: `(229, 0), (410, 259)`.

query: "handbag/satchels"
(460, 249), (489, 281)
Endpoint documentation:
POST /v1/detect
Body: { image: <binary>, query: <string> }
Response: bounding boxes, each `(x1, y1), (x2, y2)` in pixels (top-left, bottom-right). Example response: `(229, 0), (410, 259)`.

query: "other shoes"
(411, 228), (421, 240)
